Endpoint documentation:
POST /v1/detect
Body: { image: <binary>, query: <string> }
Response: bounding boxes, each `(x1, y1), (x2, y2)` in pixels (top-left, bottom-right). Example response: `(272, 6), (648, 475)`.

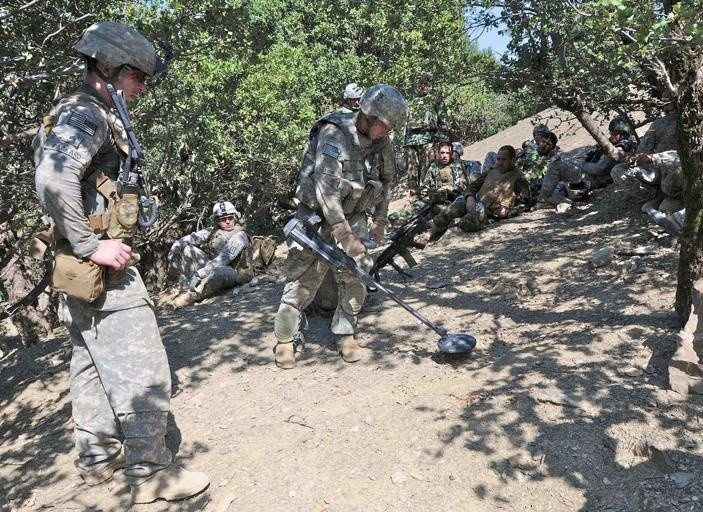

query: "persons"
(272, 84), (406, 370)
(154, 202), (254, 314)
(29, 21), (212, 503)
(384, 113), (636, 242)
(607, 87), (686, 213)
(337, 84), (364, 114)
(387, 79), (462, 203)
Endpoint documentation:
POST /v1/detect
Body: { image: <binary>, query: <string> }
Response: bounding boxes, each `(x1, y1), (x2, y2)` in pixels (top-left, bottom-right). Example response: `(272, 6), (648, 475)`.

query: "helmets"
(75, 22), (156, 76)
(533, 124), (548, 138)
(608, 118), (629, 136)
(211, 202), (241, 218)
(343, 83), (407, 130)
(452, 142), (463, 156)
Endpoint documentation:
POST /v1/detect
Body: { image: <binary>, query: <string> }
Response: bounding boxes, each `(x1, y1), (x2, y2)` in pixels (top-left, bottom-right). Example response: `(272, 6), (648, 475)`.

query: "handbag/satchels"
(50, 248), (105, 303)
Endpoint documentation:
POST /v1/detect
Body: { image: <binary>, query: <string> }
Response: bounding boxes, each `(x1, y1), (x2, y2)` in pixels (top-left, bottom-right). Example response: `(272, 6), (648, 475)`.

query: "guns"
(369, 216), (432, 292)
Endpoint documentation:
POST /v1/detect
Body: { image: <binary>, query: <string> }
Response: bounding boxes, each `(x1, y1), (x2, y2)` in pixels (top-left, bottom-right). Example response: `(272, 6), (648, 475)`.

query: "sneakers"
(275, 341), (296, 369)
(82, 444), (210, 503)
(336, 334), (362, 362)
